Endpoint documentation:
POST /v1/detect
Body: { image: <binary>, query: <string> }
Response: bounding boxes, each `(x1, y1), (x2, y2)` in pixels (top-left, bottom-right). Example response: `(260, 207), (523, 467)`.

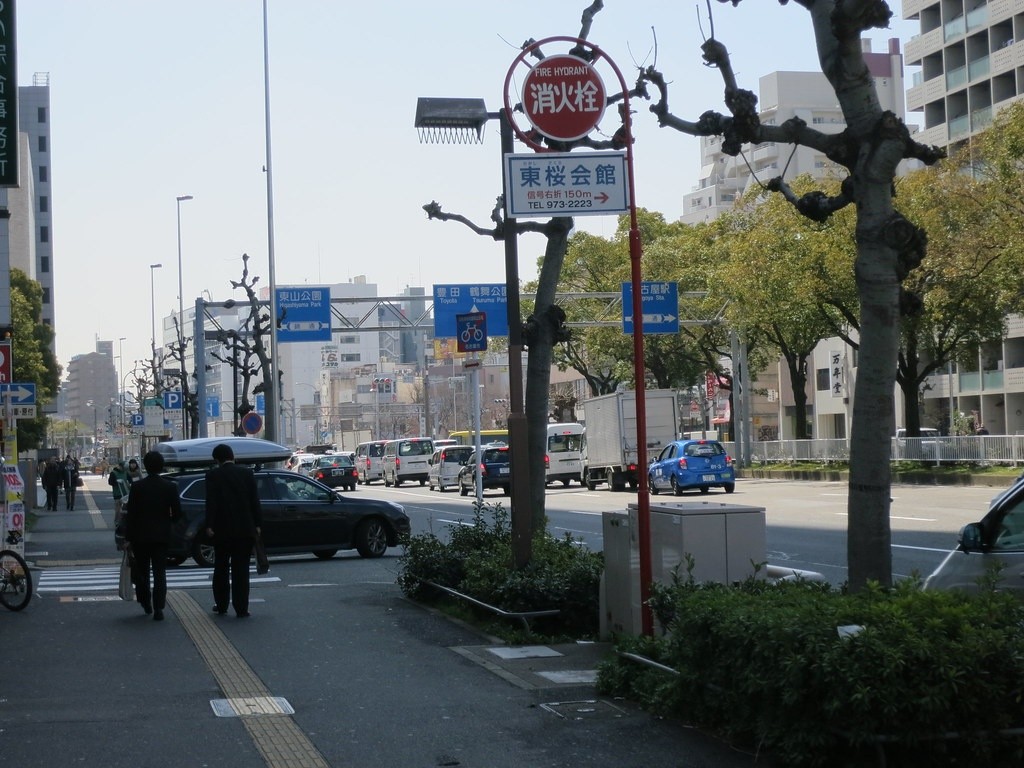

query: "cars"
(286, 450), (354, 486)
(428, 446), (474, 491)
(894, 427), (946, 457)
(307, 454), (357, 491)
(644, 441), (736, 494)
(925, 474), (1024, 596)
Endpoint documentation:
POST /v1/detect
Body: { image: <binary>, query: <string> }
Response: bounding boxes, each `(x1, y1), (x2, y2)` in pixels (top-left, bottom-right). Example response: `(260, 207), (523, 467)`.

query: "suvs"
(456, 445), (511, 496)
(155, 437), (411, 563)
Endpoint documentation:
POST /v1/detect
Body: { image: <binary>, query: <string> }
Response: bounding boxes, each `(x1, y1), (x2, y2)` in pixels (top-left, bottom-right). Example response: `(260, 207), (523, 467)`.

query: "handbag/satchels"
(119, 551), (133, 601)
(254, 533), (269, 575)
(76, 478), (83, 487)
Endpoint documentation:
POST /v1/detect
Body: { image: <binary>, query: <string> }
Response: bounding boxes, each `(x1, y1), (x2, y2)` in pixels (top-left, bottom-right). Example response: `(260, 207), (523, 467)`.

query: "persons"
(206, 444), (261, 618)
(976, 423), (989, 435)
(37, 455), (142, 526)
(113, 451), (183, 620)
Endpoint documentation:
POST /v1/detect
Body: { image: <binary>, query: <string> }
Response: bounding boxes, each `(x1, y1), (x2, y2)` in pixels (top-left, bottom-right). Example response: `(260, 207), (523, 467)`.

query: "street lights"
(120, 337), (126, 460)
(178, 194), (194, 437)
(295, 382), (319, 444)
(151, 264), (163, 397)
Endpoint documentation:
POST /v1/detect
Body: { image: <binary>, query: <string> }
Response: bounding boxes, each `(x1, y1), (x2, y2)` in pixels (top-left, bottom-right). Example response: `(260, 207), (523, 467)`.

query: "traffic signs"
(621, 283), (679, 334)
(432, 283), (509, 337)
(275, 286), (331, 343)
(502, 151), (633, 217)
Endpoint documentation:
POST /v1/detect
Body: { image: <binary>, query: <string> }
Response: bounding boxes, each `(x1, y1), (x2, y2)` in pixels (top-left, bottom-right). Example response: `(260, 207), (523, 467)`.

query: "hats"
(120, 461), (130, 469)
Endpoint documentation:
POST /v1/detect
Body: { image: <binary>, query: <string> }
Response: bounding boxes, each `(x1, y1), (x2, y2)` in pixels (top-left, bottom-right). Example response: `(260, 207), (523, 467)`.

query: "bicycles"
(0, 549), (33, 611)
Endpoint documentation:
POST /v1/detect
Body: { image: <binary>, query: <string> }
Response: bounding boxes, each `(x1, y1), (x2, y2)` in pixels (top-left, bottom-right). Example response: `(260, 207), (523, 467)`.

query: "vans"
(432, 441), (458, 448)
(381, 437), (435, 488)
(545, 423), (584, 486)
(82, 455), (94, 467)
(352, 440), (387, 485)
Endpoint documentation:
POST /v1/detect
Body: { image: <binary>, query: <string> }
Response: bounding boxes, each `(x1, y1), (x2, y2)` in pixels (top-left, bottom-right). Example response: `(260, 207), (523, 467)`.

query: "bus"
(447, 430), (510, 445)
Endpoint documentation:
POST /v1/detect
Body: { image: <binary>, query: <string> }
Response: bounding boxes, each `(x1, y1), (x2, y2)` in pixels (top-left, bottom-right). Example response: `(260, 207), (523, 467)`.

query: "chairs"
(274, 483), (288, 501)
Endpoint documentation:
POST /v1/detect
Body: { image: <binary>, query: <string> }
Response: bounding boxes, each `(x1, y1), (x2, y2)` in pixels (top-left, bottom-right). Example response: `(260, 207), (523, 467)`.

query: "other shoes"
(47, 506), (52, 511)
(53, 508), (56, 511)
(153, 609), (164, 621)
(142, 605), (154, 616)
(237, 611), (250, 618)
(70, 507), (73, 511)
(213, 604), (226, 614)
(66, 504), (71, 510)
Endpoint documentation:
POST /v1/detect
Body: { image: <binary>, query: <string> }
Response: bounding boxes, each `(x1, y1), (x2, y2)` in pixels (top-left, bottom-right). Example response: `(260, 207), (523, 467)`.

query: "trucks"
(568, 389), (679, 491)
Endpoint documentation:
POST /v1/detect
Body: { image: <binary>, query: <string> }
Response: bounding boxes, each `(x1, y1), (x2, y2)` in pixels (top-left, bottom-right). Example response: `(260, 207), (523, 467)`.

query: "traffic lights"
(373, 378), (391, 390)
